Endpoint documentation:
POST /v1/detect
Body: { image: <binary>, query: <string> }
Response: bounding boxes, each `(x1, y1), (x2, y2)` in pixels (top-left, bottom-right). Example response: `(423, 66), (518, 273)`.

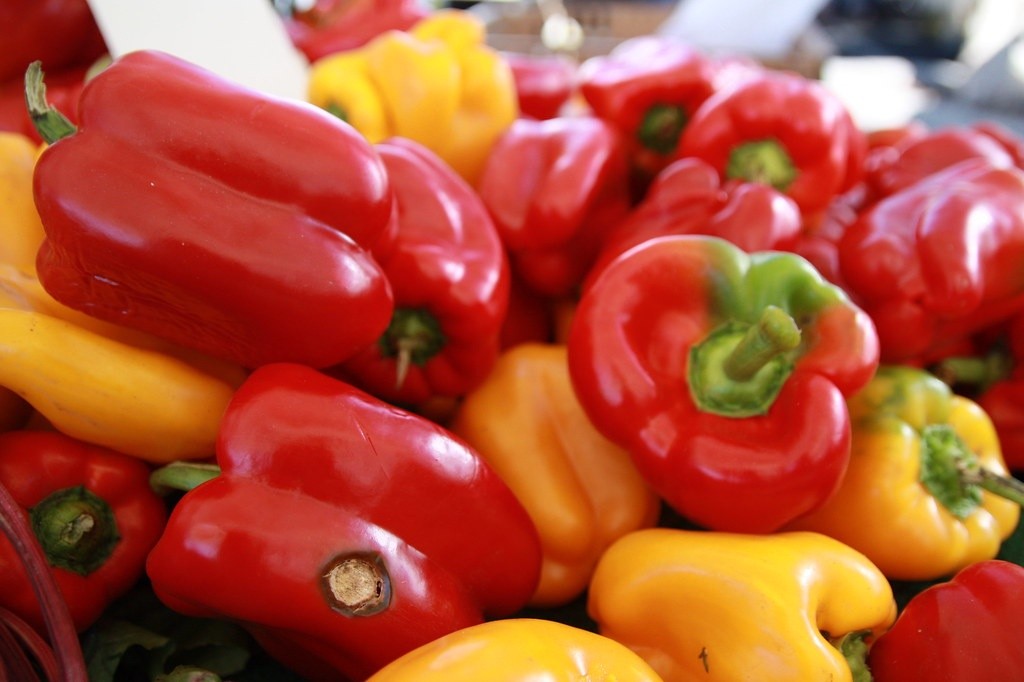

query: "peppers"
(0, 0), (1024, 682)
